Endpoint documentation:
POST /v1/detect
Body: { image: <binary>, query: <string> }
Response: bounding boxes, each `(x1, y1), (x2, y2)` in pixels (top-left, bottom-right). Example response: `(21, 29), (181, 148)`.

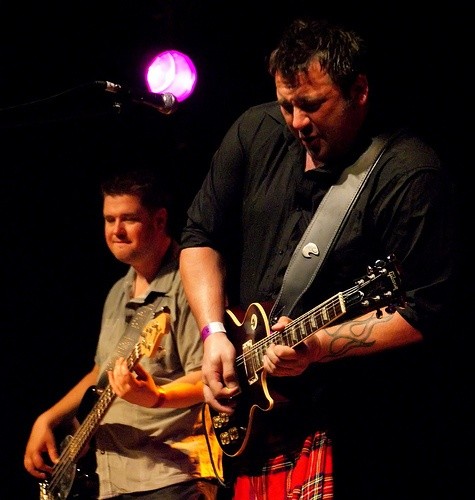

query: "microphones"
(94, 81), (178, 115)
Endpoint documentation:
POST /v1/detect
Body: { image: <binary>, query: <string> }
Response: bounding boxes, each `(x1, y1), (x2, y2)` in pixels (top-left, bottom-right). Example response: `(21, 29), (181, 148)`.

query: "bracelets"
(151, 384), (166, 408)
(199, 320), (227, 340)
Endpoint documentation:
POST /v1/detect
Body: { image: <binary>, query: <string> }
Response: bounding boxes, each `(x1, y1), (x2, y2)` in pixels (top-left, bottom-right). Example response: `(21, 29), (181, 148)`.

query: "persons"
(23, 166), (226, 500)
(175, 13), (473, 500)
